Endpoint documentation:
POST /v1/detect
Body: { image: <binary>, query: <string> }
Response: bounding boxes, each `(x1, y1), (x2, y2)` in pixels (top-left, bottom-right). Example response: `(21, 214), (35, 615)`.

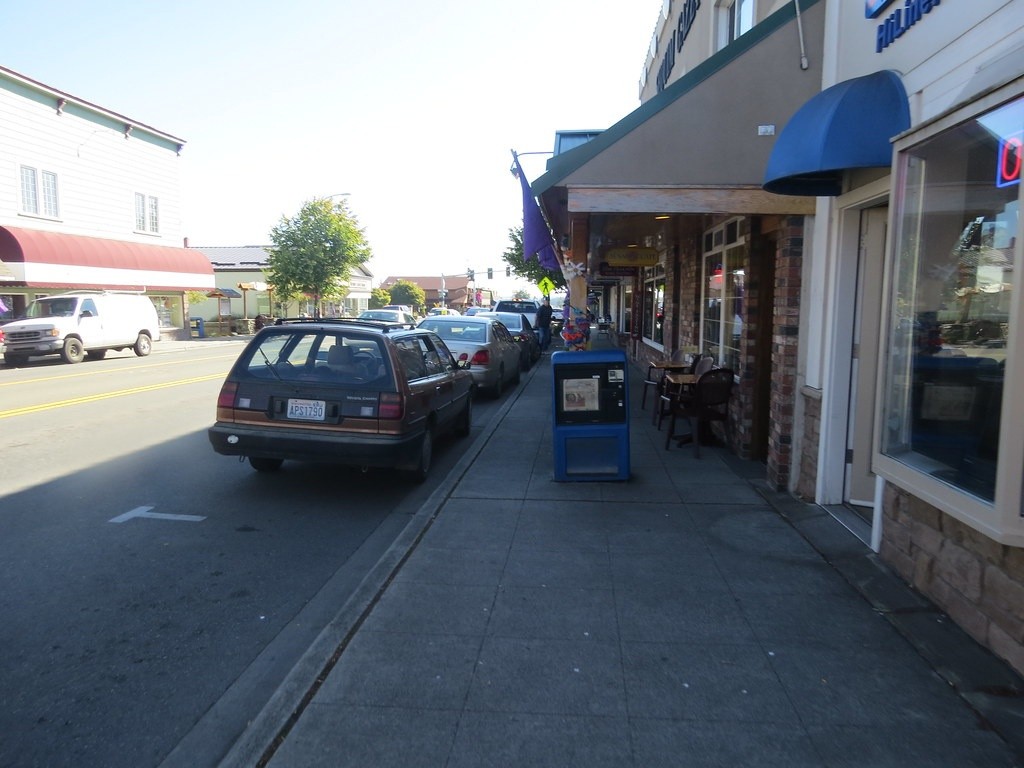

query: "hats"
(544, 297), (550, 301)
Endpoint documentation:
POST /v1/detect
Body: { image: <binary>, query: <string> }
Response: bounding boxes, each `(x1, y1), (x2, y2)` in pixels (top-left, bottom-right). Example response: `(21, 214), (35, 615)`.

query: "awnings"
(763, 68), (913, 193)
(0, 227), (216, 290)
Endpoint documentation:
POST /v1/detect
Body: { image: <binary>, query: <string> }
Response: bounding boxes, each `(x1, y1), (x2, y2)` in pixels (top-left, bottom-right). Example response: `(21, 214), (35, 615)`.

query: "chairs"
(439, 325), (452, 336)
(657, 354), (715, 435)
(664, 368), (737, 458)
(320, 344), (354, 370)
(641, 349), (684, 419)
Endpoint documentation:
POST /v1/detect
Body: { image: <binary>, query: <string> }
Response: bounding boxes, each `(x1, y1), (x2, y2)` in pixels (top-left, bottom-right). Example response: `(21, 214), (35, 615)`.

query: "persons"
(533, 295), (554, 348)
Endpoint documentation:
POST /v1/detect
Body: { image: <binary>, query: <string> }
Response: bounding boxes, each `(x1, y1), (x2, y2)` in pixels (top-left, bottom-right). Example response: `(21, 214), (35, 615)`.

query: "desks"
(665, 372), (725, 448)
(653, 360), (691, 416)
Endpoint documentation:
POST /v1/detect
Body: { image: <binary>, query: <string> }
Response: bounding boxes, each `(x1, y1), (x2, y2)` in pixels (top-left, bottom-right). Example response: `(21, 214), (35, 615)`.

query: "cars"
(209, 298), (541, 486)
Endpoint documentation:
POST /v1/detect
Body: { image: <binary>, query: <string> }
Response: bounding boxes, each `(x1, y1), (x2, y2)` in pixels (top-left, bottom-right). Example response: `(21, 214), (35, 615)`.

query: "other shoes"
(541, 351), (548, 355)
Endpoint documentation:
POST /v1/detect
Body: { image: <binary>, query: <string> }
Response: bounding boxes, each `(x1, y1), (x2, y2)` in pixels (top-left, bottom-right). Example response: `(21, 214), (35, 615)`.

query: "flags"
(533, 0), (830, 241)
(502, 150), (559, 276)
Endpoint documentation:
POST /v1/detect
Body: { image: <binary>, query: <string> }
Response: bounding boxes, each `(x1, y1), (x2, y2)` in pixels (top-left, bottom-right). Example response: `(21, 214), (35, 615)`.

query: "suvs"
(0, 286), (161, 367)
